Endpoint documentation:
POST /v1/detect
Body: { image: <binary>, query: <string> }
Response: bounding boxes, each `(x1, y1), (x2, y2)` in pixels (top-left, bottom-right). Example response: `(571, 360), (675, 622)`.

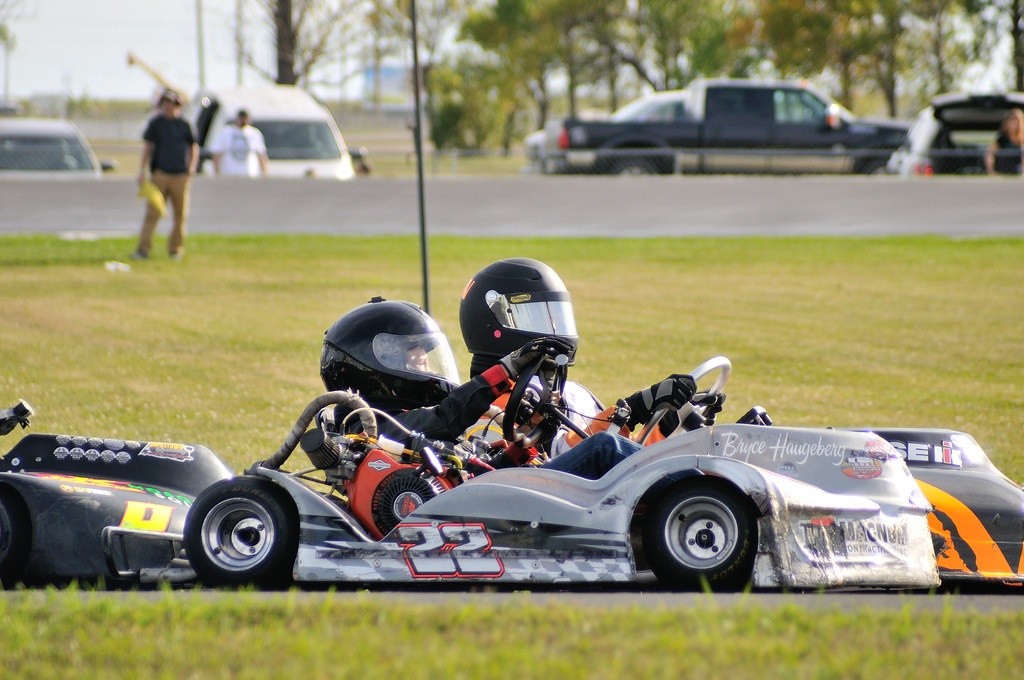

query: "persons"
(212, 110), (269, 178)
(459, 259), (725, 455)
(983, 108), (1024, 175)
(130, 90), (200, 257)
(320, 296), (646, 480)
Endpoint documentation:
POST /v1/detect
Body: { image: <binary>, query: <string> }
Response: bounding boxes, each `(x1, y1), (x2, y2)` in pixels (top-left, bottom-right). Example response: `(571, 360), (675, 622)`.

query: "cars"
(0, 117), (115, 178)
(521, 92), (696, 176)
(889, 87), (1022, 174)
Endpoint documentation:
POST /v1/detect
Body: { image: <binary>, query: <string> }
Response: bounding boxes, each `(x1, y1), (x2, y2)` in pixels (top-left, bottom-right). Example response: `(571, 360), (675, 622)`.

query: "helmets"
(319, 297), (461, 412)
(459, 257), (579, 367)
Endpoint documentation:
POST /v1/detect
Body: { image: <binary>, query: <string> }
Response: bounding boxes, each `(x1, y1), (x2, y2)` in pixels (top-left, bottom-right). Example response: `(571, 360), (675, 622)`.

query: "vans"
(184, 86), (367, 180)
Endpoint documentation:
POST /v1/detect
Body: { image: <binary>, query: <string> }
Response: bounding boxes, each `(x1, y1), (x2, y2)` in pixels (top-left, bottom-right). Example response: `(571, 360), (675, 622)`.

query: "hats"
(159, 88), (184, 106)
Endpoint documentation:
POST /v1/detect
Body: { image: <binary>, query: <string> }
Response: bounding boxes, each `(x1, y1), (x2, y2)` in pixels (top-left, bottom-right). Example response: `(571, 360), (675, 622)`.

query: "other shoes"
(172, 255), (183, 261)
(131, 252), (147, 258)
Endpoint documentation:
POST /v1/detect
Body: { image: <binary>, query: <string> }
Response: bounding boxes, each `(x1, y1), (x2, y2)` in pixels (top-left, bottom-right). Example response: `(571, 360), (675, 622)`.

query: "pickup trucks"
(541, 76), (914, 176)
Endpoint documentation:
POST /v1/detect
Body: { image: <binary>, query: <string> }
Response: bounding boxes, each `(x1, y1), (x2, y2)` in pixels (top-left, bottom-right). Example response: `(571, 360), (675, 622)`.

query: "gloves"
(499, 337), (575, 379)
(531, 396), (566, 445)
(623, 374), (697, 432)
(658, 389), (726, 437)
